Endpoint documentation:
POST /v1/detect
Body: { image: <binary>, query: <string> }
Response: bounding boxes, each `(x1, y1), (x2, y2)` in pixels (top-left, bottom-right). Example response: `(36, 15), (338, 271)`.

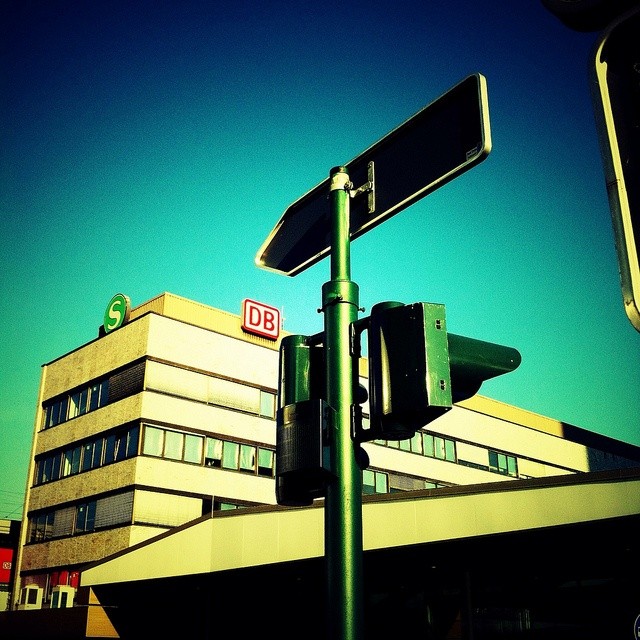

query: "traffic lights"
(366, 299), (525, 441)
